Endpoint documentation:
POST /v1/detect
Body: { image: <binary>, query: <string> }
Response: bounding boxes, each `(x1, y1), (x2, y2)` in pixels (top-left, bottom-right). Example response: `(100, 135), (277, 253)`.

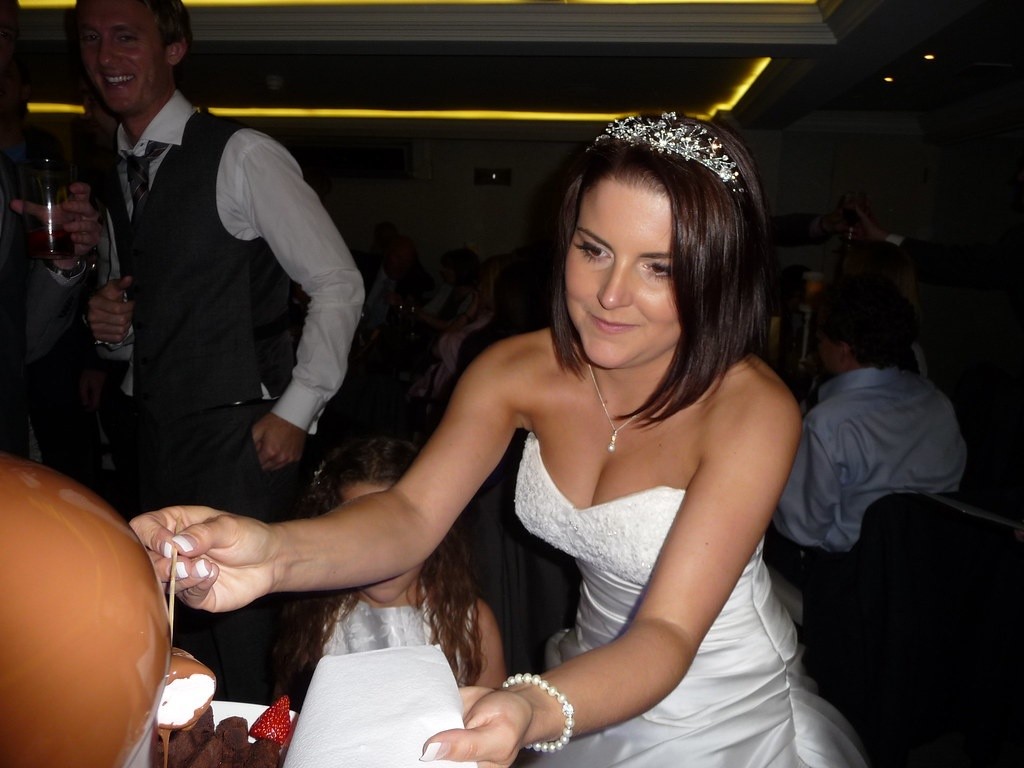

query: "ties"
(122, 140), (170, 236)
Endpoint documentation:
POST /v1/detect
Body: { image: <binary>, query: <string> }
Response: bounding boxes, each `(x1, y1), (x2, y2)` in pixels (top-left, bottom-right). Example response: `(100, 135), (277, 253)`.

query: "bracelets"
(503, 674), (576, 753)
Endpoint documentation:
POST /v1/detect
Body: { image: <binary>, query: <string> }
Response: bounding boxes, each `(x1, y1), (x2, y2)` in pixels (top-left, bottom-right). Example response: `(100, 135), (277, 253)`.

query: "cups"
(18, 156), (74, 260)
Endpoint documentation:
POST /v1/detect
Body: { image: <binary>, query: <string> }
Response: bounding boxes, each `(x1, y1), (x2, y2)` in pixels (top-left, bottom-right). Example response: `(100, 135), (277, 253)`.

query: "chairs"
(466, 486), (531, 678)
(762, 493), (1023, 768)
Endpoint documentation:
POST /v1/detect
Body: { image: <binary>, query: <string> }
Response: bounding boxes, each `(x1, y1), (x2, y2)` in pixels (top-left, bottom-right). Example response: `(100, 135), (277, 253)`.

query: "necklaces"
(588, 361), (645, 452)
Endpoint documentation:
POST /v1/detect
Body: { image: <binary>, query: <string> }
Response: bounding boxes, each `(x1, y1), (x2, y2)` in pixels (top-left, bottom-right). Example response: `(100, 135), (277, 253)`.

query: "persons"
(0, 0), (363, 703)
(127, 108), (1024, 768)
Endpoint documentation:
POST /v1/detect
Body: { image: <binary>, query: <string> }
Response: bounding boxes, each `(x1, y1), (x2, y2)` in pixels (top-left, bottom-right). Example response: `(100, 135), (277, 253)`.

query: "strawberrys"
(248, 693), (292, 746)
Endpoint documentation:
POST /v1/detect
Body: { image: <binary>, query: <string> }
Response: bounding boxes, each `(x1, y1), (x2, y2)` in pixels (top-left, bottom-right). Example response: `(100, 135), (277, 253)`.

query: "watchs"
(44, 255), (83, 279)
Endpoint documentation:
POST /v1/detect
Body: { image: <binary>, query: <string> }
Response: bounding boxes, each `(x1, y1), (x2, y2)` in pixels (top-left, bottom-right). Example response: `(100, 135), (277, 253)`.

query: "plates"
(210, 701), (299, 755)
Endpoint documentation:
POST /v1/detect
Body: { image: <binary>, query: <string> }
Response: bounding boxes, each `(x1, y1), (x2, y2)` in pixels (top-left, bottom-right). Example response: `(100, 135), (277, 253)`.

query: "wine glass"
(842, 189), (867, 240)
